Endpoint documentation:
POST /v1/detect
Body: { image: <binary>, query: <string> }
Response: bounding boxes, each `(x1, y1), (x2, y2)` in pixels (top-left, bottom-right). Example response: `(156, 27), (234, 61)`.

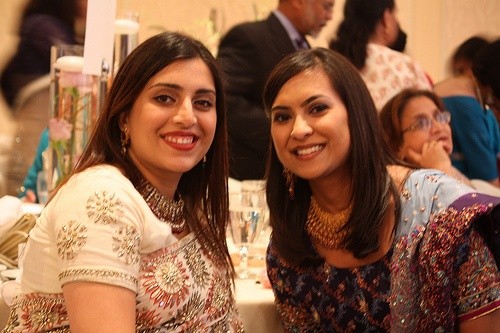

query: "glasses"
(402, 111), (451, 133)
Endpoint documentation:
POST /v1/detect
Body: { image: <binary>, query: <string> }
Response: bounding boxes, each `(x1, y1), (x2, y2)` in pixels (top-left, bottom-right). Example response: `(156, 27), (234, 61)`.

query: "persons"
(1, 31), (244, 333)
(265, 47), (500, 332)
(215, 0), (499, 191)
(0, 0), (87, 194)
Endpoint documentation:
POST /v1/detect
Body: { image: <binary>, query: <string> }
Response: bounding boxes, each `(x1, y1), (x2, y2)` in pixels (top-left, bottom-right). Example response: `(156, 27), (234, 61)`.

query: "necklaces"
(130, 171), (185, 233)
(306, 194), (354, 250)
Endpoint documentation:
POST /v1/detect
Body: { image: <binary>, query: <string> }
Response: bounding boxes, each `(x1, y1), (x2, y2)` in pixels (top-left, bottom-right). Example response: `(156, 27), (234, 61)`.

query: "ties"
(295, 39), (306, 51)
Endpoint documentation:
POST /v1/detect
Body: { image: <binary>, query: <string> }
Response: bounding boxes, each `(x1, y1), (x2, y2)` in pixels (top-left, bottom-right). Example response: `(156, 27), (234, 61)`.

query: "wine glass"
(230, 209), (265, 283)
